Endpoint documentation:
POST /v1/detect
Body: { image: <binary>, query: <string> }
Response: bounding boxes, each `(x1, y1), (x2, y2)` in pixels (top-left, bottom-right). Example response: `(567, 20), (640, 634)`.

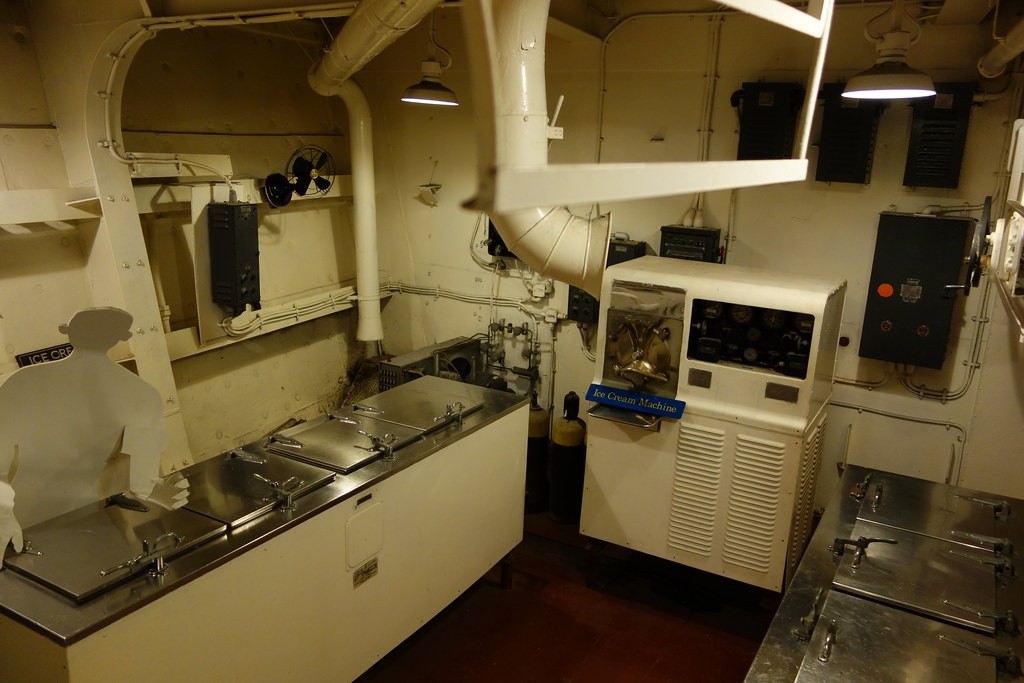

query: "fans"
(265, 144), (336, 208)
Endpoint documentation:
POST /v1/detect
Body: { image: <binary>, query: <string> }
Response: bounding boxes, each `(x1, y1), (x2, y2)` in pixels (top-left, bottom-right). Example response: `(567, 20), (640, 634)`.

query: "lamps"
(400, 10), (460, 106)
(841, 0), (936, 100)
